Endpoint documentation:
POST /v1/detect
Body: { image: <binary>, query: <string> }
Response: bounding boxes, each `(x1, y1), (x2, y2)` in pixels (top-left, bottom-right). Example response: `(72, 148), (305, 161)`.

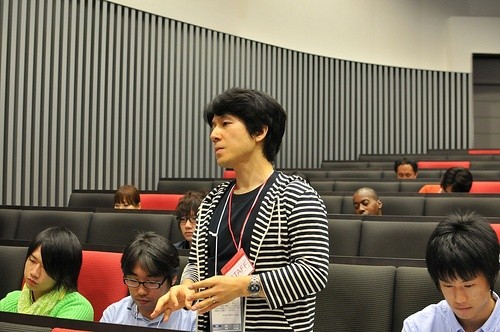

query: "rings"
(210, 296), (216, 304)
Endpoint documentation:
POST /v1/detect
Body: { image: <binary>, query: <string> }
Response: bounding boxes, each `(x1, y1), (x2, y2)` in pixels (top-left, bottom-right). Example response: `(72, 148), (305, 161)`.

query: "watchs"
(247, 275), (263, 298)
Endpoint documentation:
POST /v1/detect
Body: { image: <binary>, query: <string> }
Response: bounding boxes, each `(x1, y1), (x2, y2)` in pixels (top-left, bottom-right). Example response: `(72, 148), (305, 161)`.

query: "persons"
(99, 232), (198, 332)
(401, 211), (500, 332)
(114, 185), (142, 210)
(352, 187), (382, 217)
(150, 87), (329, 332)
(394, 158), (418, 179)
(439, 167), (473, 193)
(0, 226), (94, 321)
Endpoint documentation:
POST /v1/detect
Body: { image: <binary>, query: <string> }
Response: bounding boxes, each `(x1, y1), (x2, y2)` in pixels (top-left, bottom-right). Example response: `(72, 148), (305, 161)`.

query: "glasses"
(175, 214), (199, 225)
(121, 275), (170, 289)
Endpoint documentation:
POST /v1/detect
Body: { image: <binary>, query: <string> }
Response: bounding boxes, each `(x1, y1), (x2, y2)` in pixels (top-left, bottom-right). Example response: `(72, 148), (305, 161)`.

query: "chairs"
(0, 149), (500, 332)
